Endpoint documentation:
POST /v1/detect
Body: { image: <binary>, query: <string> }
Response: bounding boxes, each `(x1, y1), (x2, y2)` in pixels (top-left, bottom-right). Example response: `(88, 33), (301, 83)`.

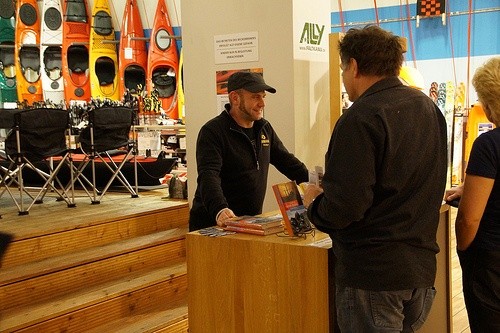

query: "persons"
(445, 56), (500, 333)
(303, 24), (448, 333)
(188, 72), (309, 231)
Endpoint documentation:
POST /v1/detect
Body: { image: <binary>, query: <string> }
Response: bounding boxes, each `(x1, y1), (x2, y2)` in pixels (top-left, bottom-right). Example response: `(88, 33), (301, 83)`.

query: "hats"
(227, 72), (276, 94)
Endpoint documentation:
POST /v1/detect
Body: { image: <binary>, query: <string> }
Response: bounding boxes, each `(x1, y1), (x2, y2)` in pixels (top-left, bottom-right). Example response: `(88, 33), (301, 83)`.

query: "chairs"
(0, 106), (139, 216)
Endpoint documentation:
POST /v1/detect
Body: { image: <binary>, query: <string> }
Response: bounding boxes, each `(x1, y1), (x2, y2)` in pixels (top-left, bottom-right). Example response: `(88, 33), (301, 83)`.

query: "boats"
(22, 0), (68, 111)
(71, 0), (122, 101)
(160, 31), (187, 134)
(128, 0), (182, 119)
(101, 0), (149, 114)
(0, 0), (18, 110)
(42, 0), (90, 112)
(0, 0), (42, 110)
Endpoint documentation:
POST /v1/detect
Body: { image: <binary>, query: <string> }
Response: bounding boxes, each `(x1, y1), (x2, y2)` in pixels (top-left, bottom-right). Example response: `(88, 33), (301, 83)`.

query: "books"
(272, 181), (305, 237)
(223, 214), (282, 230)
(225, 226), (284, 235)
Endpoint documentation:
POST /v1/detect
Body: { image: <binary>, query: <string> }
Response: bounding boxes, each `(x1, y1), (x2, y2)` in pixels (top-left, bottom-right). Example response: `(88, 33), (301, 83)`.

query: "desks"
(186, 201), (453, 333)
